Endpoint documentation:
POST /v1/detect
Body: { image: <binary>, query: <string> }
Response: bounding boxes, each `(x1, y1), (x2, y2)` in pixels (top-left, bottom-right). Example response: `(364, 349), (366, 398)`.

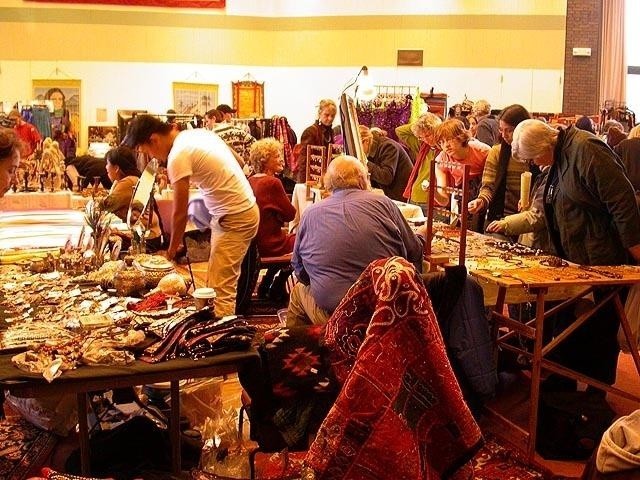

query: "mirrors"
(126, 157), (160, 229)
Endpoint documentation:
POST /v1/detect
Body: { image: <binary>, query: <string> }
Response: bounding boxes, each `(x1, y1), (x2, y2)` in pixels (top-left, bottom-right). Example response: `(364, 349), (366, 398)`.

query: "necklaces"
(504, 259), (640, 281)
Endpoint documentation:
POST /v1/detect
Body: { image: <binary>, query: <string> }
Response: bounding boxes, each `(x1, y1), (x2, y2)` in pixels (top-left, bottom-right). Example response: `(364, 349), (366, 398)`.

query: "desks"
(0, 246), (264, 479)
(467, 266), (640, 462)
(289, 185), (594, 407)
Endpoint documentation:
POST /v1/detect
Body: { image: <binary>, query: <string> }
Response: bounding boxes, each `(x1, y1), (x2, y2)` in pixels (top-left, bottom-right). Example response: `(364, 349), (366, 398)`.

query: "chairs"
(234, 254), (484, 478)
(1, 183), (640, 317)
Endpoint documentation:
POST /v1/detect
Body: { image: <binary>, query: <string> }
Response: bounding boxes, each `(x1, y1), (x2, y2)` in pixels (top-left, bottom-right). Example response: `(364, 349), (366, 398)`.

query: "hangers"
(604, 100), (626, 109)
(230, 116), (286, 126)
(372, 84), (420, 98)
(142, 112), (193, 121)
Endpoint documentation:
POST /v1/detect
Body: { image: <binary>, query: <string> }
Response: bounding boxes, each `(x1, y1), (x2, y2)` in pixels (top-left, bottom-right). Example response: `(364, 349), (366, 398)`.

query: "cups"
(192, 288), (216, 311)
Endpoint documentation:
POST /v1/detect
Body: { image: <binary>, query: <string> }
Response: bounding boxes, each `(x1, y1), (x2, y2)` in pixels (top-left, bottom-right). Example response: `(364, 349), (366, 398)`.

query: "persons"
(298, 98), (338, 185)
(131, 202), (144, 224)
(42, 87), (76, 158)
(484, 163), (549, 254)
(101, 146), (163, 253)
(121, 113), (260, 320)
(287, 154), (423, 327)
(205, 109), (256, 176)
(394, 112), (442, 214)
(448, 100), (639, 196)
(510, 117), (640, 402)
(245, 135), (300, 306)
(165, 110), (177, 125)
(62, 154), (112, 191)
(8, 109), (42, 170)
(0, 125), (23, 199)
(424, 118), (492, 234)
(217, 105), (237, 123)
(467, 104), (540, 242)
(358, 125), (414, 205)
